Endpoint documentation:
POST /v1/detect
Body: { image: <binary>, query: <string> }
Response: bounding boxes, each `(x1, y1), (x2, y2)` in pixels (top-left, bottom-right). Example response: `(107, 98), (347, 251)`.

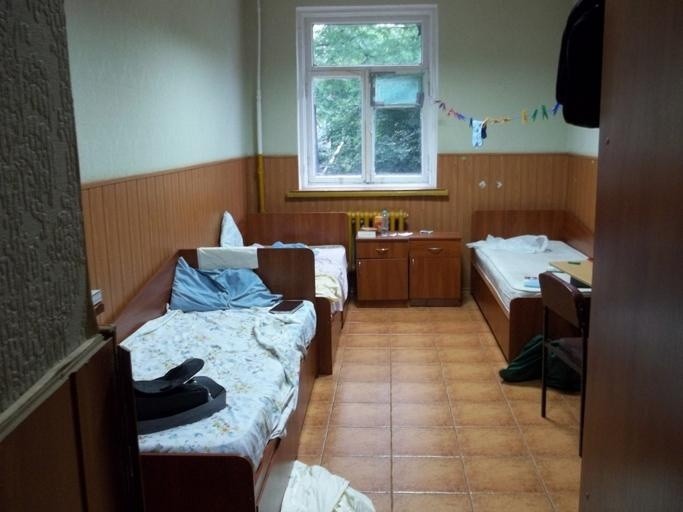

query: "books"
(91, 288), (102, 306)
(357, 225), (377, 239)
(269, 299), (304, 315)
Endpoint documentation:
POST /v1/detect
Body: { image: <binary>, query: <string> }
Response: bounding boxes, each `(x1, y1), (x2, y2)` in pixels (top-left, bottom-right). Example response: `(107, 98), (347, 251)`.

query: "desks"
(548, 257), (592, 286)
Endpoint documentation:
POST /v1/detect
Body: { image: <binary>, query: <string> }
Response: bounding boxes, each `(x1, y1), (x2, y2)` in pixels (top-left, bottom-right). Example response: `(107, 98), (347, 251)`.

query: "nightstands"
(356, 230), (461, 305)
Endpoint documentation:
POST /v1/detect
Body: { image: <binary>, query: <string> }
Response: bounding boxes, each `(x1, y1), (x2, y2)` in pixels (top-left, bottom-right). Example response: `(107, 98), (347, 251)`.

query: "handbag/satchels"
(131, 358), (225, 435)
(499, 335), (582, 394)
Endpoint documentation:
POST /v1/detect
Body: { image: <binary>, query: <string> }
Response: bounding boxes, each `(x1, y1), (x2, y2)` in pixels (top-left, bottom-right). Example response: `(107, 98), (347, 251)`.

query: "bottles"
(380, 208), (388, 237)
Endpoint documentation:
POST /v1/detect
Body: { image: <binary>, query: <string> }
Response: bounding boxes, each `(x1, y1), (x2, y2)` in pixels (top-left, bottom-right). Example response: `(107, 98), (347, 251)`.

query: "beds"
(471, 211), (595, 364)
(220, 209), (350, 377)
(105, 247), (318, 510)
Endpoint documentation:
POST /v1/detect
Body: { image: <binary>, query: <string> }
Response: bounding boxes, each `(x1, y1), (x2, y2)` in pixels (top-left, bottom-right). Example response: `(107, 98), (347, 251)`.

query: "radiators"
(346, 210), (409, 271)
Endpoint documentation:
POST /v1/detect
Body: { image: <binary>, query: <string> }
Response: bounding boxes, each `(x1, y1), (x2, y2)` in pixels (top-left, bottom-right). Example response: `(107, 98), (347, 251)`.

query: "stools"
(539, 268), (591, 458)
